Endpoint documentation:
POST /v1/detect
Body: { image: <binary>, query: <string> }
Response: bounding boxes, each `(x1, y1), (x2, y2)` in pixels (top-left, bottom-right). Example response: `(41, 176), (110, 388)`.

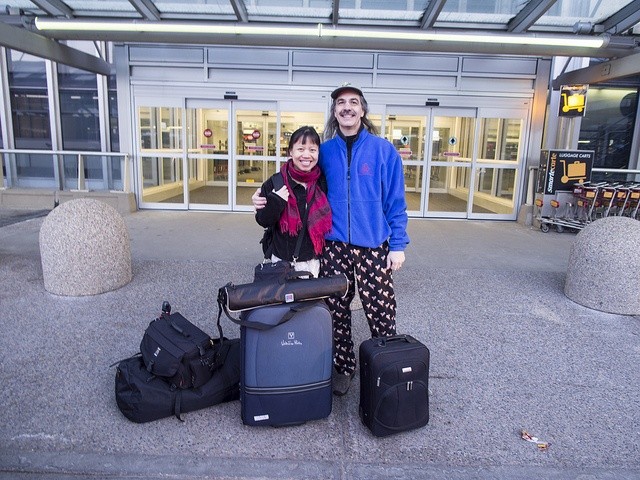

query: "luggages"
(238, 298), (334, 428)
(359, 334), (430, 437)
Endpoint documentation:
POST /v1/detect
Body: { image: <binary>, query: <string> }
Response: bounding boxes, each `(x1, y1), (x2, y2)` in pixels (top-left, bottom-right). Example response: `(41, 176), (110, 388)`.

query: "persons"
(319, 86), (410, 395)
(255, 125), (334, 279)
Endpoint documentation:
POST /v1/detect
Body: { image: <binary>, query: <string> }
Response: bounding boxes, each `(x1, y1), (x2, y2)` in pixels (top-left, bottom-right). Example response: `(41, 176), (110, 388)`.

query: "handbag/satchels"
(253, 255), (299, 284)
(141, 300), (218, 391)
(216, 271), (349, 313)
(109, 338), (240, 423)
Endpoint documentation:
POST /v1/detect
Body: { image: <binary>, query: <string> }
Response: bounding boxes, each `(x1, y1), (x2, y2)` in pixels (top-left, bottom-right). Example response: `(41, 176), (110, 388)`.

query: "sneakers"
(332, 367), (357, 396)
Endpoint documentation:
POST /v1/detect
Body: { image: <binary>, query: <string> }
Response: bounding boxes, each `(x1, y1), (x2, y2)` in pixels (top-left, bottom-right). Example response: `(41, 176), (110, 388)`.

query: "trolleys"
(535, 180), (640, 232)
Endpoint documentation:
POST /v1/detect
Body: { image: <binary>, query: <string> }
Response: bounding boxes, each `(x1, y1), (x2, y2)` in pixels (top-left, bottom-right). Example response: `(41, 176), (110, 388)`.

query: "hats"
(331, 82), (363, 99)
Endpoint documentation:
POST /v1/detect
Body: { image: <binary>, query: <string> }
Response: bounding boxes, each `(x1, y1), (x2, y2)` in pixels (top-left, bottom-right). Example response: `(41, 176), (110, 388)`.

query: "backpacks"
(260, 171), (327, 260)
